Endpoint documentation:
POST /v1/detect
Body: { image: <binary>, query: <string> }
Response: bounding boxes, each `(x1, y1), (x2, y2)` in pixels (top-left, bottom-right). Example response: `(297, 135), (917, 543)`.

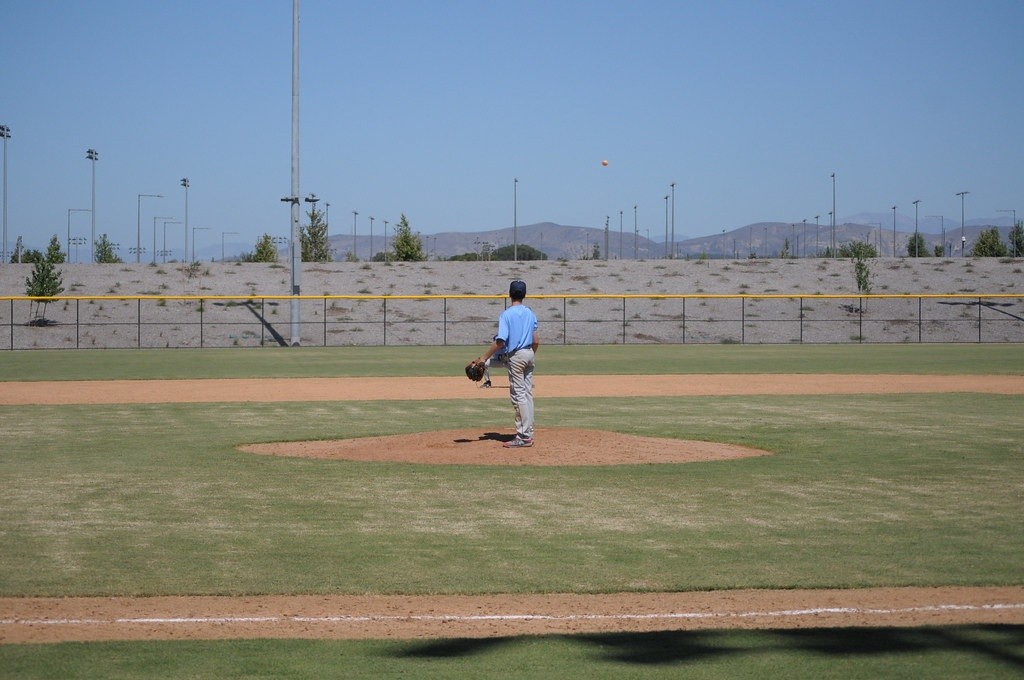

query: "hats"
(510, 281), (526, 299)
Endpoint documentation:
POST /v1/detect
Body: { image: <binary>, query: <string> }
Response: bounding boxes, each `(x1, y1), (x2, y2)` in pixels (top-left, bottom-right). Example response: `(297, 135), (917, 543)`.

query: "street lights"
(371, 217), (374, 262)
(814, 215), (820, 251)
(913, 200), (920, 257)
(802, 219), (807, 257)
(830, 172), (836, 257)
(514, 178), (519, 259)
(434, 237), (437, 255)
(620, 211), (623, 259)
(86, 148), (99, 264)
(664, 196), (669, 258)
(352, 211), (359, 257)
(326, 203), (330, 239)
(723, 230), (726, 258)
(305, 192), (320, 227)
(670, 183), (676, 259)
(180, 177), (189, 262)
(633, 205), (636, 259)
(426, 235), (429, 255)
(384, 220), (390, 261)
(892, 206), (897, 257)
(765, 228), (768, 255)
(0, 124), (12, 264)
(956, 191), (968, 257)
(792, 224), (794, 248)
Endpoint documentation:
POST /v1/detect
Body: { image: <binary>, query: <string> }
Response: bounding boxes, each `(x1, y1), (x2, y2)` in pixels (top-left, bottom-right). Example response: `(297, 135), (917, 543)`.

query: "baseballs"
(602, 160), (608, 166)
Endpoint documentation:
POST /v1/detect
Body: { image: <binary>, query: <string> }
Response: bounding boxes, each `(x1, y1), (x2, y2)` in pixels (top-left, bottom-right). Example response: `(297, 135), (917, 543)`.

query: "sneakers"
(503, 437), (534, 448)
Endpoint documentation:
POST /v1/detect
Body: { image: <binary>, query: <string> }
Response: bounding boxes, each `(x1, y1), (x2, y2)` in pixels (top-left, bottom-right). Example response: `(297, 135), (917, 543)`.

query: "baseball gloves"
(464, 357), (486, 382)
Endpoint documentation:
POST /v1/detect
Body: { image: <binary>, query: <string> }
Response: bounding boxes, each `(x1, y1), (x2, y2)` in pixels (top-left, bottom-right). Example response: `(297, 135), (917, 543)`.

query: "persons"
(465, 281), (539, 448)
(480, 335), (508, 389)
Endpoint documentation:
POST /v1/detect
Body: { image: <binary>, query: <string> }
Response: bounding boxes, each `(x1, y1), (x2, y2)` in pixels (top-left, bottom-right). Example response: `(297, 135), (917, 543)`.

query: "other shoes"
(481, 380), (491, 387)
